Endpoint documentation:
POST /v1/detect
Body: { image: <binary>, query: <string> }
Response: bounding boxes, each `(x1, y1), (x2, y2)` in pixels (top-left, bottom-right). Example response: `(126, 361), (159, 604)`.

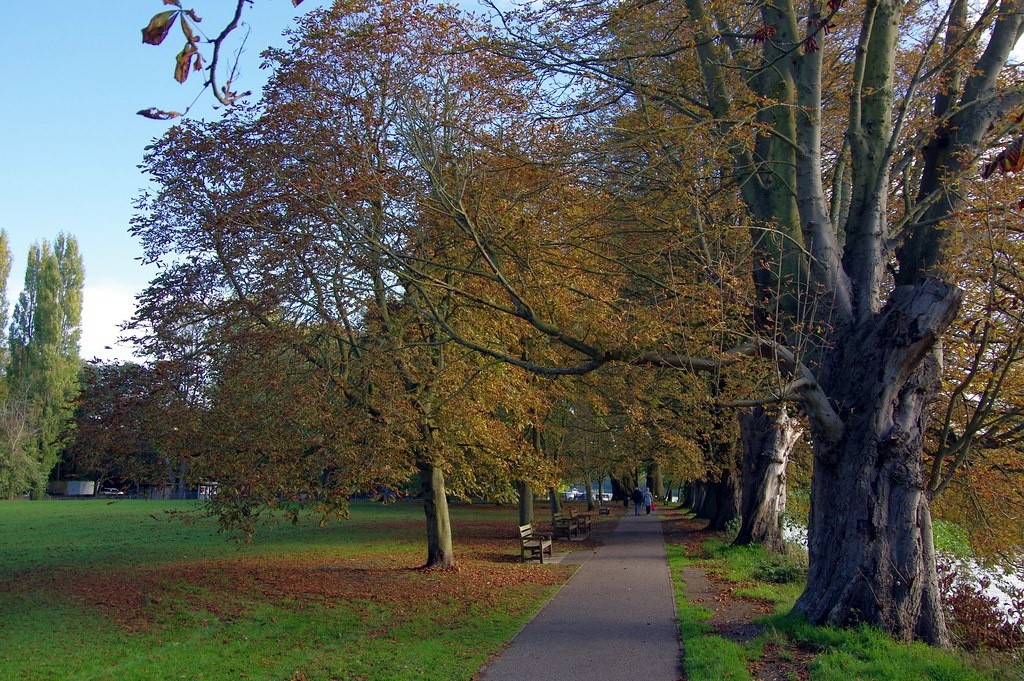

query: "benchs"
(598, 500), (610, 516)
(517, 523), (553, 564)
(552, 511), (578, 542)
(597, 495), (609, 501)
(569, 509), (591, 534)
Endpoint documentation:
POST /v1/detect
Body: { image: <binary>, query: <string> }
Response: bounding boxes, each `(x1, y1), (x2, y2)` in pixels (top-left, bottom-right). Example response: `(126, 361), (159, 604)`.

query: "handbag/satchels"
(652, 502), (655, 511)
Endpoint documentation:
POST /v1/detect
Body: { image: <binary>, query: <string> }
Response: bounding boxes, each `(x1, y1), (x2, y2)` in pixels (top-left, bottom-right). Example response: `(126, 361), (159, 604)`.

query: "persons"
(642, 487), (653, 517)
(632, 487), (643, 516)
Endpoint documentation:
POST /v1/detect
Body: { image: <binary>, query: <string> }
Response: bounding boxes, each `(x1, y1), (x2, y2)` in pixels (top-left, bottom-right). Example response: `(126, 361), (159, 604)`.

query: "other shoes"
(648, 514), (650, 516)
(635, 514), (640, 516)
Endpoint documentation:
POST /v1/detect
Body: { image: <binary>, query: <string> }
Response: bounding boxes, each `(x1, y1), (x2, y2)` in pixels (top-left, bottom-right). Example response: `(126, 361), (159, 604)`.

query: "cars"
(562, 490), (614, 502)
